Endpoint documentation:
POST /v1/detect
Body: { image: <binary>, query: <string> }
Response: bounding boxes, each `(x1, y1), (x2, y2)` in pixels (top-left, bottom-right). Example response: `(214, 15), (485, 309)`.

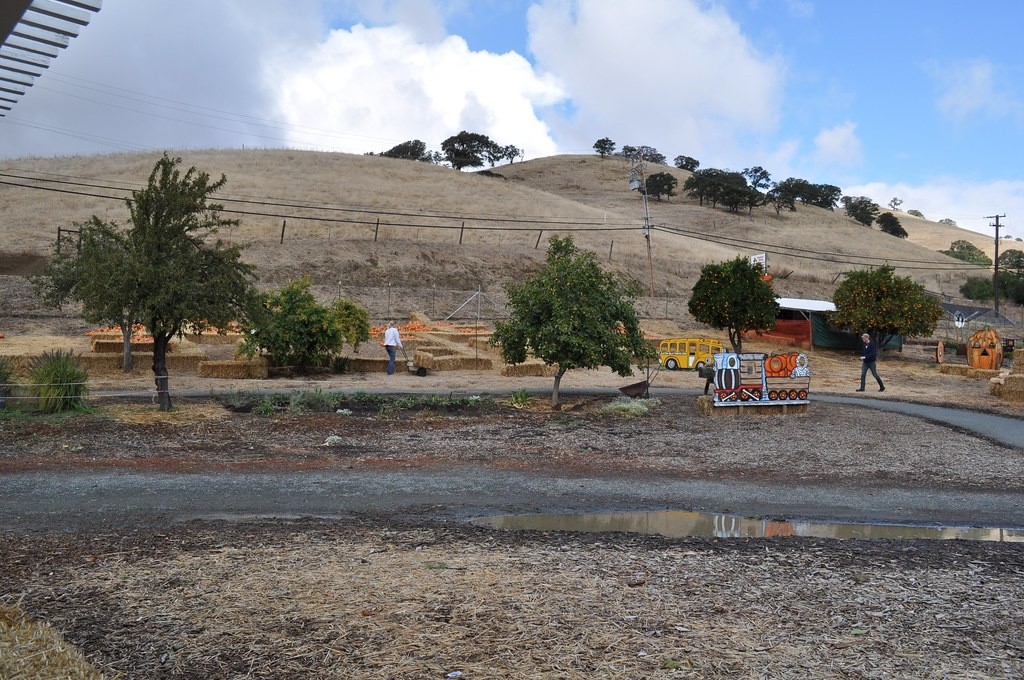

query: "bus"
(659, 338), (724, 371)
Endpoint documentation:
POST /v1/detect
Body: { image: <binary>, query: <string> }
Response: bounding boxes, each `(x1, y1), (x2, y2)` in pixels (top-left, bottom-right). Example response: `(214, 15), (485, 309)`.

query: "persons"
(703, 359), (717, 395)
(855, 333), (886, 393)
(384, 320), (403, 375)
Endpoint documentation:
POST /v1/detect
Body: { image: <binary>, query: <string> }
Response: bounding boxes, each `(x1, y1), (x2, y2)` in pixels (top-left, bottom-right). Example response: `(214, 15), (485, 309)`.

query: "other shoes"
(856, 388), (864, 391)
(878, 387), (885, 391)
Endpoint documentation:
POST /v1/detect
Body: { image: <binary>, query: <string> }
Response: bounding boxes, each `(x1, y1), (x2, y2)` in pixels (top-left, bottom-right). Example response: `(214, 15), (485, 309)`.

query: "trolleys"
(398, 344), (428, 376)
(618, 368), (660, 399)
